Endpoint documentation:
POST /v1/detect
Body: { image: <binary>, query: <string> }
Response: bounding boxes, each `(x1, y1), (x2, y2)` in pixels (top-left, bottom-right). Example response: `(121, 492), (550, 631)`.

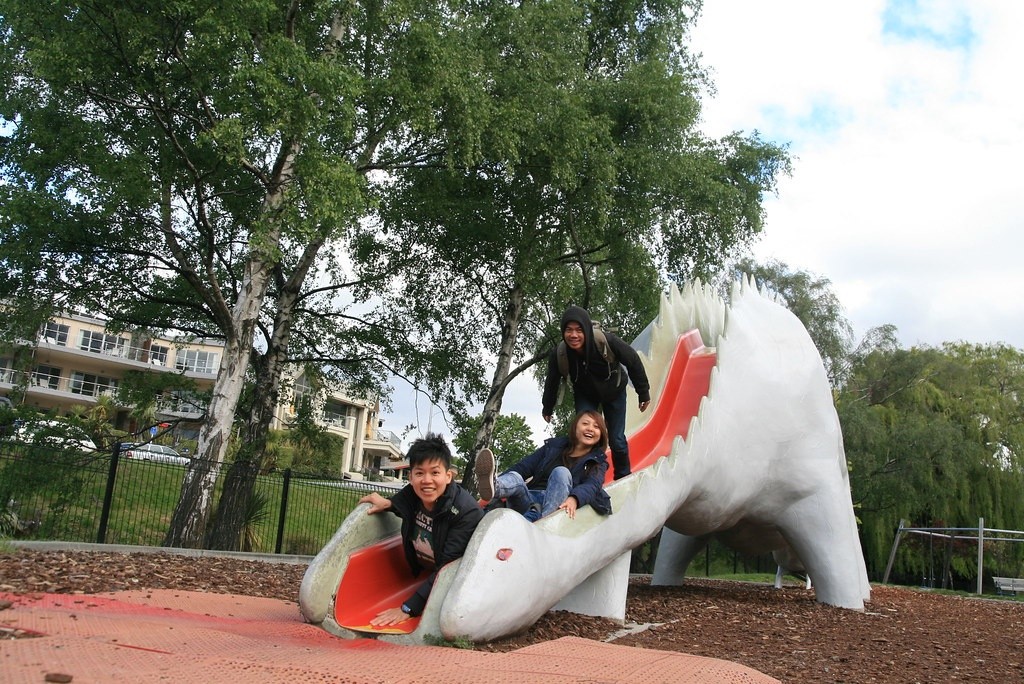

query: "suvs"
(119, 443), (190, 466)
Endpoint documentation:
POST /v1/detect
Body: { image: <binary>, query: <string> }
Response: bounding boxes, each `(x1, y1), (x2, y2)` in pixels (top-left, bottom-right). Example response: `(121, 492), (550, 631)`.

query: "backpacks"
(557, 320), (622, 405)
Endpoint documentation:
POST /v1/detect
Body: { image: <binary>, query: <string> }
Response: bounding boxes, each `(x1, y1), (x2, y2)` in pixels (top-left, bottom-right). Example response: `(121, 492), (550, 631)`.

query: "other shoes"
(476, 449), (495, 501)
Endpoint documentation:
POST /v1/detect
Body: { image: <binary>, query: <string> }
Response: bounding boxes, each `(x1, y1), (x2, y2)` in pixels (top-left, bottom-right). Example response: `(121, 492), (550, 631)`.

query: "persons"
(541, 305), (651, 481)
(358, 436), (543, 626)
(473, 407), (615, 524)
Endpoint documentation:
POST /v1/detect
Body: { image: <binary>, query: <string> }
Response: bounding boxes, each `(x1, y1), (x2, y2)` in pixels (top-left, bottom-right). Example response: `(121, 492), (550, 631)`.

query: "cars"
(13, 419), (97, 453)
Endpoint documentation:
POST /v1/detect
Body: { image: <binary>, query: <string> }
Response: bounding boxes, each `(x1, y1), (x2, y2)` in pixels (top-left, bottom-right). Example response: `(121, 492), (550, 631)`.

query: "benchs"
(992, 577), (1024, 596)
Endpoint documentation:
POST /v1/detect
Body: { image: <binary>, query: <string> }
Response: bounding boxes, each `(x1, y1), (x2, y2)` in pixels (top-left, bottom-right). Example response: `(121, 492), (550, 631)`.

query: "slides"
(298, 328), (719, 647)
